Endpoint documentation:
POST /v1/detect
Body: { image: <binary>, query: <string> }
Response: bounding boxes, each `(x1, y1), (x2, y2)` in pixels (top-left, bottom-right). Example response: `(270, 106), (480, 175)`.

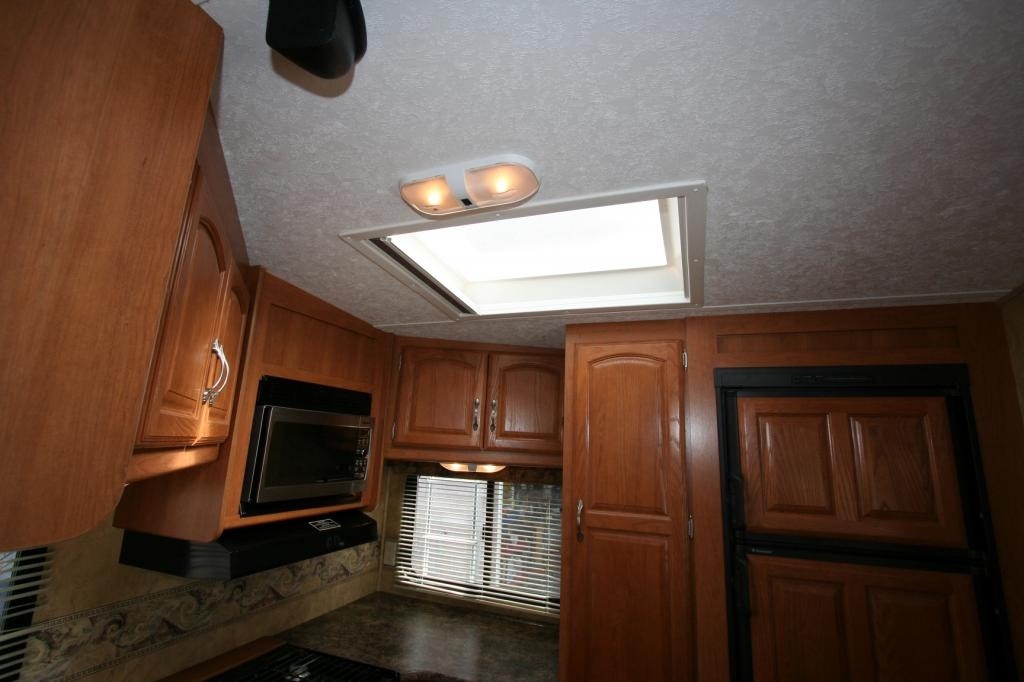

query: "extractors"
(118, 504), (378, 580)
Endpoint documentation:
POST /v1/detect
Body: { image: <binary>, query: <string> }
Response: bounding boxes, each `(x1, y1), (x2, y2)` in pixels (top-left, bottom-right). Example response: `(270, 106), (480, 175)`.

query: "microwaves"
(237, 405), (376, 509)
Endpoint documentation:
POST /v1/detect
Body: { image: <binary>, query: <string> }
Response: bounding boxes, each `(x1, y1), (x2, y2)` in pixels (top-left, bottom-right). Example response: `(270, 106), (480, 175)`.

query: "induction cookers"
(196, 642), (399, 682)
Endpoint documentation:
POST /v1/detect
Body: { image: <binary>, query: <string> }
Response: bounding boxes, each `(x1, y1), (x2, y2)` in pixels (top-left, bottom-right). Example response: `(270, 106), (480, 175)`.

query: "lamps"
(440, 463), (505, 474)
(398, 156), (541, 218)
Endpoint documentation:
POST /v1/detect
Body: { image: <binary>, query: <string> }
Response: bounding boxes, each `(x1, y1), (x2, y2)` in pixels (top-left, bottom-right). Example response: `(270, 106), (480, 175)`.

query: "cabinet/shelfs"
(128, 107), (256, 489)
(731, 388), (972, 553)
(389, 333), (564, 469)
(562, 319), (695, 682)
(746, 550), (986, 681)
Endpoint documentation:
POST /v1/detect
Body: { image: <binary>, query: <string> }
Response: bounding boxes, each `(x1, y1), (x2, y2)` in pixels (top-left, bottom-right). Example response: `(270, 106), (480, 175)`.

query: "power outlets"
(382, 541), (396, 566)
